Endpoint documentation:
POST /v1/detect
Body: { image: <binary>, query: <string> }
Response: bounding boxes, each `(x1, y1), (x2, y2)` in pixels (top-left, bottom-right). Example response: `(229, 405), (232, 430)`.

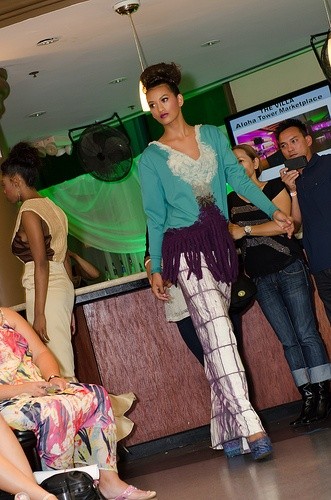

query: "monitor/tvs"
(223, 79), (331, 182)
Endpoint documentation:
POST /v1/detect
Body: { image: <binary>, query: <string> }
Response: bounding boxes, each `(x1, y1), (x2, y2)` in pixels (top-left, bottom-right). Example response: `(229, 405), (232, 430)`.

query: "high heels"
(222, 439), (249, 460)
(245, 432), (274, 460)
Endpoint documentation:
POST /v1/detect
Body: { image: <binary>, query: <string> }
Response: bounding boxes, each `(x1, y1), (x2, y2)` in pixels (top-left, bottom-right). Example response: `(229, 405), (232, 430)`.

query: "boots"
(291, 380), (330, 428)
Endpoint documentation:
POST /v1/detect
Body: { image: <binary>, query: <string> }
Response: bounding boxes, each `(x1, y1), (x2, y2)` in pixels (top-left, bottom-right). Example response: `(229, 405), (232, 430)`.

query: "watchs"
(243, 225), (252, 236)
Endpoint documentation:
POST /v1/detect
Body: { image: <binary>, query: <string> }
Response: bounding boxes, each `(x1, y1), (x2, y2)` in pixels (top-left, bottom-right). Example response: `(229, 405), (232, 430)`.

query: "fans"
(68, 111), (133, 182)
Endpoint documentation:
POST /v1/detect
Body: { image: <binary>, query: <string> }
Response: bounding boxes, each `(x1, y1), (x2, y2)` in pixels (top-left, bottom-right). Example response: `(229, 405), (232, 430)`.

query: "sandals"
(92, 478), (158, 499)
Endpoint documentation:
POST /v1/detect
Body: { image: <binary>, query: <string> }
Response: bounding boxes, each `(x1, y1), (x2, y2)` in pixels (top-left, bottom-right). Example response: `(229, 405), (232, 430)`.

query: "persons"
(274, 118), (331, 324)
(143, 222), (272, 438)
(226, 144), (331, 427)
(0, 304), (157, 500)
(67, 248), (101, 289)
(137, 60), (295, 461)
(0, 141), (76, 379)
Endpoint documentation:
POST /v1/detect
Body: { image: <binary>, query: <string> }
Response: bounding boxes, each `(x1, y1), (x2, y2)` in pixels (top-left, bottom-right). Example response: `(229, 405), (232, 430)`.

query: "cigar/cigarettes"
(283, 171), (287, 175)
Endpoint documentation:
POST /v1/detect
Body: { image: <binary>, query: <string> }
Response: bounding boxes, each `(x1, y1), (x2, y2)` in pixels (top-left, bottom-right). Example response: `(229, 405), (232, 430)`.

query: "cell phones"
(284, 155), (308, 172)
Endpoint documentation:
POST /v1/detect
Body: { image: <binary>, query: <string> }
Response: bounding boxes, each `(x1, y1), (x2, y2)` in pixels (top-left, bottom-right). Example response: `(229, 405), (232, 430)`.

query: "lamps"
(127, 13), (151, 111)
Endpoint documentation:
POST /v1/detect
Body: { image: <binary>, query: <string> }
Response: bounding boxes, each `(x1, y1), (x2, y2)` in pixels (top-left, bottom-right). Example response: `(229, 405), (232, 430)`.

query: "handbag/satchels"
(229, 282), (260, 314)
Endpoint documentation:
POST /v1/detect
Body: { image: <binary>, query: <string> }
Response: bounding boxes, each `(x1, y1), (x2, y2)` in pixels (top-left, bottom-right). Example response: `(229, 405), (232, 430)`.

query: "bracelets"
(290, 191), (298, 197)
(47, 375), (60, 382)
(144, 258), (152, 267)
